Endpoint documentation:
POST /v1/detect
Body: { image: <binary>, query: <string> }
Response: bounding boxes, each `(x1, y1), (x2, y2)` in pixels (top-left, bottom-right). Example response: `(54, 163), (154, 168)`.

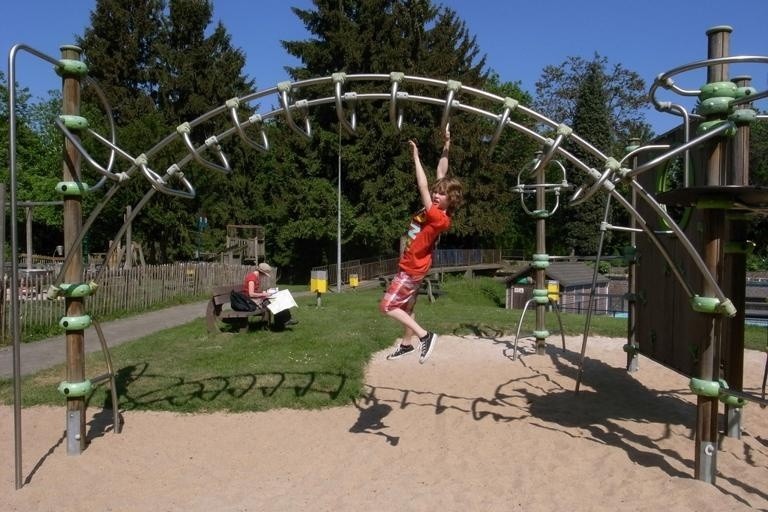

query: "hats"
(256, 262), (271, 277)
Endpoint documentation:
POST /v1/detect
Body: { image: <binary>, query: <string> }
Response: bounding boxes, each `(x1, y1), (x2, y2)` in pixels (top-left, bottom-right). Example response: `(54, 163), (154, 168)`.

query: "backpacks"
(231, 289), (256, 312)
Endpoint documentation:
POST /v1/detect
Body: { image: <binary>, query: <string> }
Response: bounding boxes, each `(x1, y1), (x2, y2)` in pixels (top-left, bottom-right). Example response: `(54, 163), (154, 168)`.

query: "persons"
(243, 261), (298, 332)
(377, 113), (464, 366)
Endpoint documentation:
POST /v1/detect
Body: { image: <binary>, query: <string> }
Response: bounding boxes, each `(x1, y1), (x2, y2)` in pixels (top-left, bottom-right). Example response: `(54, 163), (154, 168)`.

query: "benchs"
(379, 278), (448, 302)
(206, 284), (267, 336)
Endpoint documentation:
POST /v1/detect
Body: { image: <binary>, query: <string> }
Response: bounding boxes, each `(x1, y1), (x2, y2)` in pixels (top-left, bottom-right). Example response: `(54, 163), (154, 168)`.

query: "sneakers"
(387, 344), (416, 360)
(418, 333), (437, 364)
(284, 319), (298, 325)
